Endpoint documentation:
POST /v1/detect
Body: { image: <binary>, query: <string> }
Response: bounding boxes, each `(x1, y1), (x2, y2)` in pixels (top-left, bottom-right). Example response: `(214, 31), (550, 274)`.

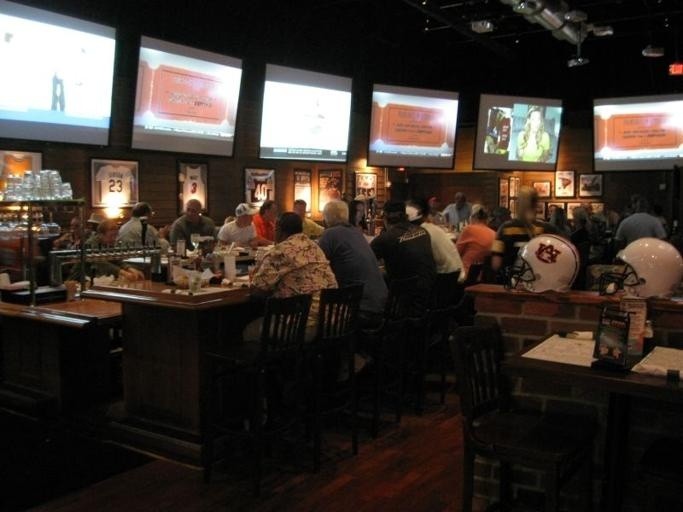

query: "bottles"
(643, 320), (654, 352)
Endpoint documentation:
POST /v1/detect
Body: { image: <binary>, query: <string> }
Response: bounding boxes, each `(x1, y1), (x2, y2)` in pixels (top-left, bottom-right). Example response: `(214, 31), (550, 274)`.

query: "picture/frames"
(497, 170), (604, 221)
(87, 150), (378, 222)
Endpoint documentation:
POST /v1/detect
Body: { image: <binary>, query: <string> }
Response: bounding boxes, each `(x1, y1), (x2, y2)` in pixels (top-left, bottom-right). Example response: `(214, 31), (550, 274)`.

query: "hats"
(406, 205), (423, 223)
(235, 203), (260, 216)
(87, 213), (104, 224)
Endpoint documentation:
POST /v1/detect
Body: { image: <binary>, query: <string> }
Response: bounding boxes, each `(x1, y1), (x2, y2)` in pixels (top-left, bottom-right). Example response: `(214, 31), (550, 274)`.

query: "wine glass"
(1, 204), (62, 242)
(190, 233), (200, 257)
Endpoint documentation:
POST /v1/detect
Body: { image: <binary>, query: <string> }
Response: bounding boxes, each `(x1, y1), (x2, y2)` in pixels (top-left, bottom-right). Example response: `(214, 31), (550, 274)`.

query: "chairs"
(192, 257), (491, 489)
(446, 321), (597, 511)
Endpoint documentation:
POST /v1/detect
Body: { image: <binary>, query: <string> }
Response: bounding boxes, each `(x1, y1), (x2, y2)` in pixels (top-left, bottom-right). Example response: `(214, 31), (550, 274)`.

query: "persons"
(516, 106), (554, 161)
(482, 111), (513, 156)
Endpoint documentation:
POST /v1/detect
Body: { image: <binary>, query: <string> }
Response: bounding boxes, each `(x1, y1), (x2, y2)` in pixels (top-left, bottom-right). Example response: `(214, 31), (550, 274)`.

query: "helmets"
(599, 238), (682, 300)
(504, 234), (580, 293)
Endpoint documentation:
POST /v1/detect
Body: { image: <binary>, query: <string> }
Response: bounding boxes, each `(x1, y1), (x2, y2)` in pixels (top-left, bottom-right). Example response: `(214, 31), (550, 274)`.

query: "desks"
(520, 328), (683, 512)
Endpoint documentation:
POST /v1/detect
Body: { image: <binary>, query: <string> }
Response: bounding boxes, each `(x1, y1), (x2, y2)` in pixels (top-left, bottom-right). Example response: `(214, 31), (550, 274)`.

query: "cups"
(176, 239), (186, 257)
(223, 256), (235, 278)
(188, 271), (202, 293)
(4, 170), (73, 203)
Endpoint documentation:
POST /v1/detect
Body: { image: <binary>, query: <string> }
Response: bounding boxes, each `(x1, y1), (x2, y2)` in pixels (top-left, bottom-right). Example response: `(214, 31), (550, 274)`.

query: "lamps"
(503, 0), (613, 70)
(469, 17), (494, 35)
(642, 43), (665, 58)
